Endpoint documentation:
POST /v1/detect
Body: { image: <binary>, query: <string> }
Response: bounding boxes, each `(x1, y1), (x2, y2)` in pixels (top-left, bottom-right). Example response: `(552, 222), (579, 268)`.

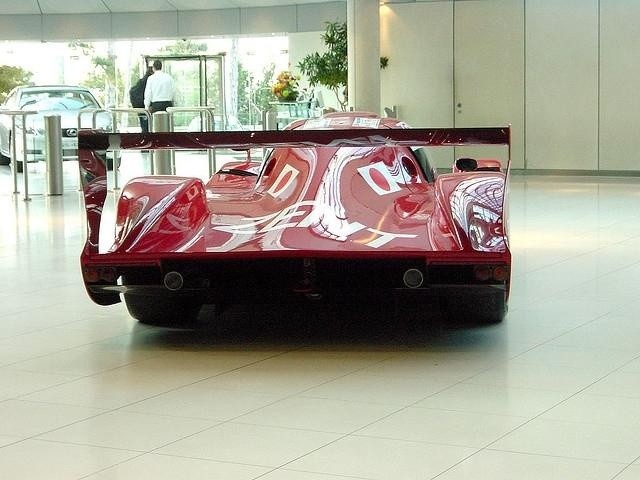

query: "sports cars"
(77, 112), (512, 329)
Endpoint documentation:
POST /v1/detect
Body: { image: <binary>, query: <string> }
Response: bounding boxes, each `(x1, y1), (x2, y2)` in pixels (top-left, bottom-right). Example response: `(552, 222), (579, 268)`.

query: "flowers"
(271, 70), (301, 102)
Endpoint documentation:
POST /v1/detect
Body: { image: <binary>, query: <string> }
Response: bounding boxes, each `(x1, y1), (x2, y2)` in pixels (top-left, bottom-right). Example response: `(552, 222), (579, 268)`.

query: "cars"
(0, 84), (121, 172)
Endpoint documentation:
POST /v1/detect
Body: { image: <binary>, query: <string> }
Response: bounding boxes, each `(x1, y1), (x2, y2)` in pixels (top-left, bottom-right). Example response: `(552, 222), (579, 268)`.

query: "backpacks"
(129, 79), (147, 108)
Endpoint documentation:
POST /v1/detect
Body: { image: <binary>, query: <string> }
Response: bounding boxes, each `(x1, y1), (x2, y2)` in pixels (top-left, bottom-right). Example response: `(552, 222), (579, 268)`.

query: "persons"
(130, 67), (154, 152)
(143, 60), (175, 111)
